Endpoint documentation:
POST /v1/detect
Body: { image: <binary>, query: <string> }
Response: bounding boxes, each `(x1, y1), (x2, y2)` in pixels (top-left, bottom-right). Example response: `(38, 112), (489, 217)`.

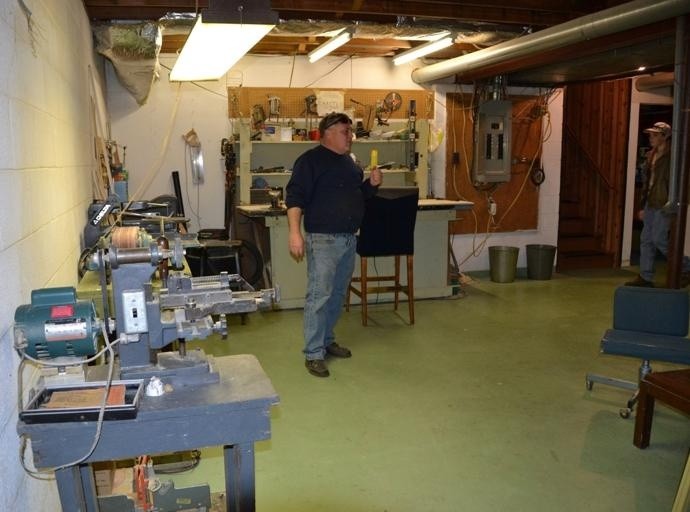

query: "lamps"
(168, 0), (280, 83)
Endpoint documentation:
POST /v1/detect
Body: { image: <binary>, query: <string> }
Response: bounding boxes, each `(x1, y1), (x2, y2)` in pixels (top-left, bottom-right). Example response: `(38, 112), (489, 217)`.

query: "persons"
(623, 121), (676, 286)
(285, 111), (383, 377)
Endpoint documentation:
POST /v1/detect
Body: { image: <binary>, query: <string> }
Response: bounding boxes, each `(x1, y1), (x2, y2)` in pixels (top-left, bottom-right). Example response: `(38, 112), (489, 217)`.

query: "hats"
(642, 121), (672, 136)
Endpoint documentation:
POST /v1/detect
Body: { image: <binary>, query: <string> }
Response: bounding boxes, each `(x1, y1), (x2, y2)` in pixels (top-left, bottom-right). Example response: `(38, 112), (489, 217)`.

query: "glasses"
(325, 115), (354, 131)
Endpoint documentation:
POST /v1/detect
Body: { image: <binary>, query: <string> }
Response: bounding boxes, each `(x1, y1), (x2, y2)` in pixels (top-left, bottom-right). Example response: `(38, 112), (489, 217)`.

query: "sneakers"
(624, 275), (654, 287)
(325, 341), (353, 358)
(304, 356), (330, 378)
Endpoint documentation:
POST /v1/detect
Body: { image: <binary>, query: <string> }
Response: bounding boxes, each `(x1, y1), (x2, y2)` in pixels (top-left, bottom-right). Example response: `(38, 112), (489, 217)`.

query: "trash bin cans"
(488, 246), (520, 283)
(526, 245), (556, 281)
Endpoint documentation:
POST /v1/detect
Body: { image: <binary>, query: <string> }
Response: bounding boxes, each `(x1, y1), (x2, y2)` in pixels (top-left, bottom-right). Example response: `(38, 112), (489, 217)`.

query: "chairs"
(585, 284), (690, 419)
(345, 186), (419, 327)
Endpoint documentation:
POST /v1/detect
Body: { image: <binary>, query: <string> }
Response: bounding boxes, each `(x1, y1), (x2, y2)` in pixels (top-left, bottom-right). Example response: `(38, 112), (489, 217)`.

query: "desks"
(17, 353), (280, 512)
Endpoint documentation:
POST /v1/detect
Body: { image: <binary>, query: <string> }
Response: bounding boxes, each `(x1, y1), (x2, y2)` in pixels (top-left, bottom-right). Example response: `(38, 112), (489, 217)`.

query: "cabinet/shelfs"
(232, 118), (429, 206)
